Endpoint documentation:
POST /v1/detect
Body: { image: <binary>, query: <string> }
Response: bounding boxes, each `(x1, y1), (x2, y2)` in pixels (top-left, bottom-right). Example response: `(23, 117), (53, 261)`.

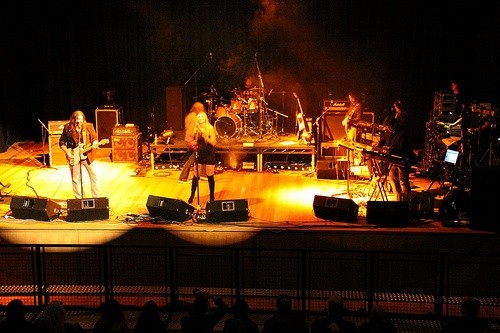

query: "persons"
(383, 80), (493, 195)
(342, 90), (363, 166)
(241, 77), (259, 98)
(179, 102), (207, 184)
(188, 112), (216, 203)
(59, 110), (102, 198)
(0, 291), (495, 333)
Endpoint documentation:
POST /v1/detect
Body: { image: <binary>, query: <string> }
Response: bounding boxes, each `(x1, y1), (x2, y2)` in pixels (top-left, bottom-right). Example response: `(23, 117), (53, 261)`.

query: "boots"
(188, 175), (200, 204)
(207, 175), (215, 201)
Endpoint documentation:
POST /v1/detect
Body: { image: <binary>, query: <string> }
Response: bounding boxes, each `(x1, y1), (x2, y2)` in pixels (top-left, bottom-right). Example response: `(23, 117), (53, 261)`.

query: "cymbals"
(214, 97), (230, 102)
(248, 87), (265, 90)
(227, 89), (243, 92)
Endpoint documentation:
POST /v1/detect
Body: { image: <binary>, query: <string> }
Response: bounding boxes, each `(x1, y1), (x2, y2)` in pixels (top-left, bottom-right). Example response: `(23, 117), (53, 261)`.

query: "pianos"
(338, 137), (416, 201)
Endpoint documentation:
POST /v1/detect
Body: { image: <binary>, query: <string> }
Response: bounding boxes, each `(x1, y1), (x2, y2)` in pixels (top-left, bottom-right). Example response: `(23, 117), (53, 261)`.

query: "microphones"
(210, 51), (213, 62)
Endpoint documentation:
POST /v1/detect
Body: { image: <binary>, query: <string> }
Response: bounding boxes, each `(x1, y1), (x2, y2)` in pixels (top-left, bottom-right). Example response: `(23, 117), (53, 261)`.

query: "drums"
(240, 108), (253, 117)
(214, 104), (229, 119)
(247, 98), (258, 114)
(230, 98), (244, 114)
(214, 113), (242, 140)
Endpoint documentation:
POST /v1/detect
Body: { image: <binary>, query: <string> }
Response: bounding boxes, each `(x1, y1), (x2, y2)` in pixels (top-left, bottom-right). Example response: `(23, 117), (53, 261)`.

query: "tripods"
(235, 104), (279, 139)
(478, 143), (499, 166)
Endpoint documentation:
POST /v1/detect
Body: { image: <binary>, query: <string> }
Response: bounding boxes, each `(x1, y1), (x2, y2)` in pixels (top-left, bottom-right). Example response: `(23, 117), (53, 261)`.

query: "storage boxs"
(95, 107), (124, 149)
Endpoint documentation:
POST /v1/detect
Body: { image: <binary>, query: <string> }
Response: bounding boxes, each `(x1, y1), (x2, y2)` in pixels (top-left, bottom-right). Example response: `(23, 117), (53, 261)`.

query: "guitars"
(66, 138), (110, 165)
(292, 91), (310, 132)
(344, 103), (359, 130)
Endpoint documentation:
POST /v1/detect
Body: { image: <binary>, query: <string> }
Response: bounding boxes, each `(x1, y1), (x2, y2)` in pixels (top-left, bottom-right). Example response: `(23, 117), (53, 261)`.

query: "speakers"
(166, 86), (186, 130)
(48, 135), (70, 167)
(10, 196), (62, 220)
(321, 112), (374, 145)
(399, 194), (435, 218)
(146, 195), (196, 220)
(204, 199), (249, 221)
(67, 197), (110, 221)
(313, 194), (359, 222)
(317, 160), (346, 179)
(366, 201), (411, 225)
(111, 132), (142, 163)
